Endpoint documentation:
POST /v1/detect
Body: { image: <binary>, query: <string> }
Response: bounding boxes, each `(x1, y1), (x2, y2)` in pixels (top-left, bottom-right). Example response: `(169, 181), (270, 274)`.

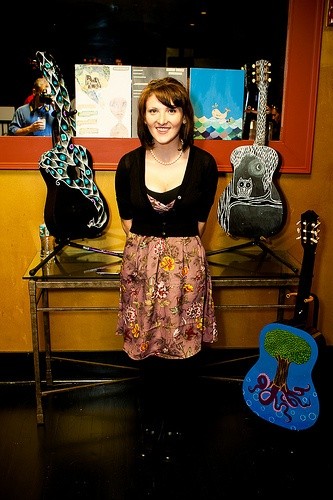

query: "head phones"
(29, 102), (49, 116)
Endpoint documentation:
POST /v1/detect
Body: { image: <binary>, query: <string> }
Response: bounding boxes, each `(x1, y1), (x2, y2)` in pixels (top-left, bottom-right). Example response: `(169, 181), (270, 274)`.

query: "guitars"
(242, 210), (326, 430)
(36, 51), (109, 241)
(217, 60), (288, 239)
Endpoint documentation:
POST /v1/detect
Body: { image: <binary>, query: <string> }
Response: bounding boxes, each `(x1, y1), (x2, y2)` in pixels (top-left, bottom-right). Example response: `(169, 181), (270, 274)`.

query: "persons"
(115, 77), (218, 465)
(272, 105), (281, 124)
(8, 78), (53, 136)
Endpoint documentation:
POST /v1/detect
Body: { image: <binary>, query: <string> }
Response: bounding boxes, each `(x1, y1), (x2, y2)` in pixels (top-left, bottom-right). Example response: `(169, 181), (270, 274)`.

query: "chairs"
(0, 119), (13, 136)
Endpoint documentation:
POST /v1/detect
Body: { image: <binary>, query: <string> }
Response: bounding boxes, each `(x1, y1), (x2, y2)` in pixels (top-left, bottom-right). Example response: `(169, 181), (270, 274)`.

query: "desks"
(21, 248), (302, 426)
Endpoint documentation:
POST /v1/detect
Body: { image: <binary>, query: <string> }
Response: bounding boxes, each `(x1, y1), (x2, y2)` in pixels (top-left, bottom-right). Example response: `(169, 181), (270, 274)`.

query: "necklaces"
(149, 139), (183, 165)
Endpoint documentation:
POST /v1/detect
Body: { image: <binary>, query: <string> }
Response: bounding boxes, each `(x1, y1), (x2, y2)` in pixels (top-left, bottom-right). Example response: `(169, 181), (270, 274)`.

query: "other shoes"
(139, 421), (197, 460)
